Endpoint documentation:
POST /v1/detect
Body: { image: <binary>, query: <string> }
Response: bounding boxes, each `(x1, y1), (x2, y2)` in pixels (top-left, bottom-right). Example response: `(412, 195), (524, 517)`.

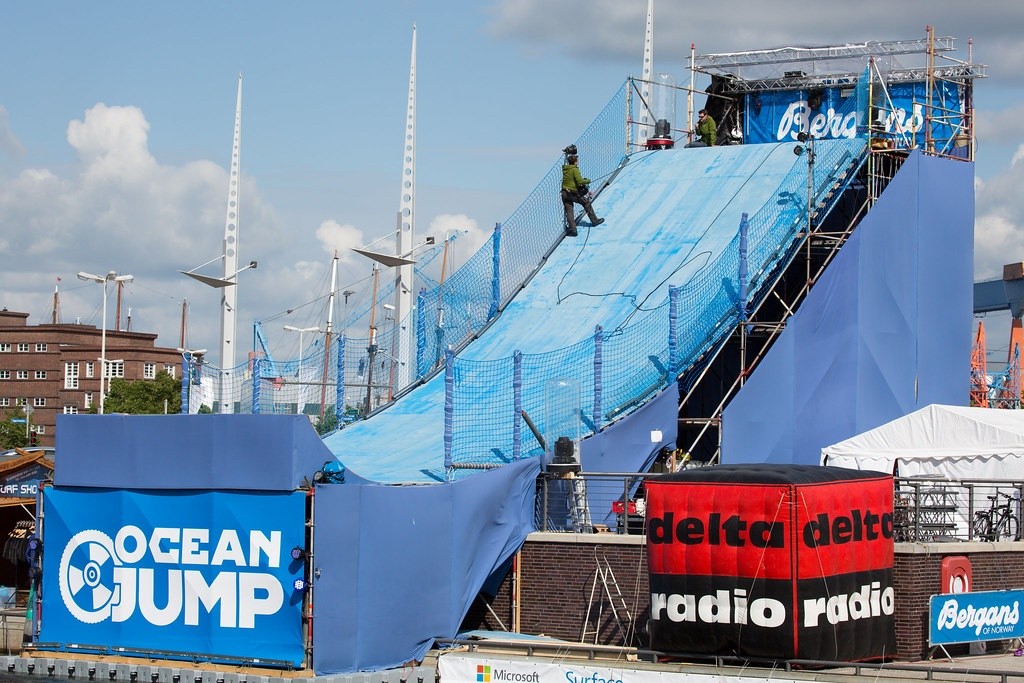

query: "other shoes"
(593, 218), (604, 227)
(572, 232), (578, 236)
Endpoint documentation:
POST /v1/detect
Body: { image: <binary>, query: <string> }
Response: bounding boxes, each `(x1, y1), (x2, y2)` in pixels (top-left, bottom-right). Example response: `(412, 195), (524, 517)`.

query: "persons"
(561, 156), (604, 237)
(684, 109), (716, 148)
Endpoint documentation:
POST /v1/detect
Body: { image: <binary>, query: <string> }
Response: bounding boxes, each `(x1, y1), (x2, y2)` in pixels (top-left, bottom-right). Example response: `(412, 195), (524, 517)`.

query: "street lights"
(177, 73), (258, 416)
(178, 348), (208, 412)
(350, 20), (437, 400)
(284, 325), (320, 415)
(78, 270), (134, 414)
(97, 358), (123, 397)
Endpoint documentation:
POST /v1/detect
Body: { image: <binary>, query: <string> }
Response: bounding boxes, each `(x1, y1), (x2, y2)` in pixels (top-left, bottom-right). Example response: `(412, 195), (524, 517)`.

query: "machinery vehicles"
(253, 230), (479, 378)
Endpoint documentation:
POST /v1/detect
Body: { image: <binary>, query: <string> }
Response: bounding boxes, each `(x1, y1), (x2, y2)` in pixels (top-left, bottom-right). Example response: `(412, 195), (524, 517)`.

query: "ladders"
(580, 544), (642, 647)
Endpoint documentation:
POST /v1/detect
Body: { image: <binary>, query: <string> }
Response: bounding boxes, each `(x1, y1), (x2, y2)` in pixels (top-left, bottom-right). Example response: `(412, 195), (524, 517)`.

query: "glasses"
(699, 114), (706, 119)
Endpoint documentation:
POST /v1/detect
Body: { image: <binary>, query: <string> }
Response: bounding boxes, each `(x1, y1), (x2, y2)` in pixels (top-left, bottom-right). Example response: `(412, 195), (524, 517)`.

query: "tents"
(820, 404), (1024, 542)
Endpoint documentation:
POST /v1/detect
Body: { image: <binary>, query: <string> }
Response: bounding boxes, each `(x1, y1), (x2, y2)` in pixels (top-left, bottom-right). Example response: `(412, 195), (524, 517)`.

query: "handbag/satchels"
(578, 186), (588, 196)
